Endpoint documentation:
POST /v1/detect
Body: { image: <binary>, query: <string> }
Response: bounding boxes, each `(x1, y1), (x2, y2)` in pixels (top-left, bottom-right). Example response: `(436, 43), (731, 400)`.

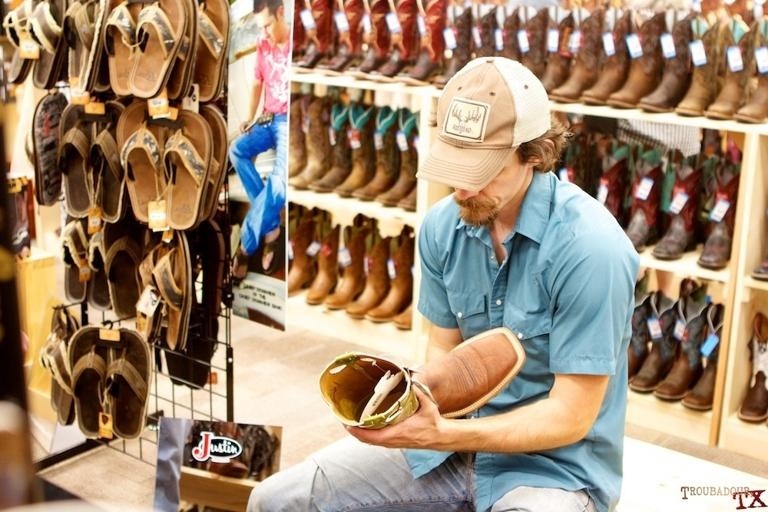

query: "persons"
(245, 57), (640, 512)
(228, 0), (292, 288)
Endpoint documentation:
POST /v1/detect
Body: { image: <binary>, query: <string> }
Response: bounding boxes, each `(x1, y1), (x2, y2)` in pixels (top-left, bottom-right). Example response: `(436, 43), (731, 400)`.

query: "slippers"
(151, 231), (192, 352)
(262, 226), (285, 275)
(2, 0), (39, 84)
(127, 0), (188, 99)
(74, 0), (110, 93)
(48, 311), (79, 425)
(116, 102), (165, 225)
(57, 103), (94, 218)
(186, 303), (218, 390)
(25, 91), (68, 206)
(200, 105), (230, 222)
(62, 214), (89, 304)
(138, 241), (169, 343)
(197, 220), (225, 319)
(163, 110), (214, 231)
(63, 0), (88, 91)
(89, 101), (128, 224)
(28, 0), (69, 89)
(231, 241), (249, 282)
(88, 231), (112, 311)
(105, 328), (152, 442)
(141, 227), (163, 260)
(193, 0), (231, 105)
(39, 306), (65, 412)
(68, 325), (107, 437)
(102, 221), (145, 320)
(167, 0), (199, 102)
(104, 1), (152, 97)
(165, 350), (186, 386)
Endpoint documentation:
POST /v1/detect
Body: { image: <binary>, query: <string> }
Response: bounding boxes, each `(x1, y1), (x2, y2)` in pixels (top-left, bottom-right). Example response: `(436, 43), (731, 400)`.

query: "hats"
(416, 57), (551, 193)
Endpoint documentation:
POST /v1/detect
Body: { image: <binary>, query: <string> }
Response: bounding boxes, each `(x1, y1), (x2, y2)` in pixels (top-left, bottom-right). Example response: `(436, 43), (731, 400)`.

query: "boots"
(678, 279), (707, 305)
(500, 9), (523, 65)
(397, 186), (416, 213)
(297, 0), (340, 68)
(549, 9), (603, 103)
(365, 234), (415, 322)
(597, 141), (630, 228)
(288, 94), (329, 190)
(703, 22), (759, 120)
(307, 105), (348, 193)
(345, 229), (390, 318)
(289, 202), (307, 241)
(319, 326), (527, 430)
(308, 207), (331, 243)
(379, 0), (421, 78)
(540, 12), (574, 94)
(751, 259), (768, 279)
(674, 19), (720, 116)
(651, 167), (704, 260)
(733, 33), (768, 124)
(390, 225), (414, 255)
(307, 223), (340, 303)
(738, 312), (768, 421)
(343, 213), (382, 254)
(654, 294), (713, 400)
(522, 8), (549, 78)
(606, 11), (668, 109)
(289, 93), (304, 177)
(288, 221), (317, 293)
(566, 131), (742, 222)
(351, 105), (398, 201)
(579, 10), (632, 106)
(328, 0), (365, 72)
(697, 164), (740, 269)
(565, 134), (603, 200)
(629, 290), (679, 391)
(334, 103), (378, 197)
(374, 108), (420, 206)
(626, 291), (655, 378)
(359, 1), (391, 73)
(681, 304), (725, 410)
(476, 5), (500, 58)
(634, 268), (651, 303)
(625, 144), (664, 250)
(409, 0), (448, 81)
(326, 225), (367, 310)
(392, 304), (413, 329)
(637, 12), (695, 113)
(435, 6), (472, 89)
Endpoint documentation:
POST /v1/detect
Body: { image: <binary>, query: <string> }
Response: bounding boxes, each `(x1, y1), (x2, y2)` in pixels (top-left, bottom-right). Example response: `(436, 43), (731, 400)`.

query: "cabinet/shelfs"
(288, 0), (768, 479)
